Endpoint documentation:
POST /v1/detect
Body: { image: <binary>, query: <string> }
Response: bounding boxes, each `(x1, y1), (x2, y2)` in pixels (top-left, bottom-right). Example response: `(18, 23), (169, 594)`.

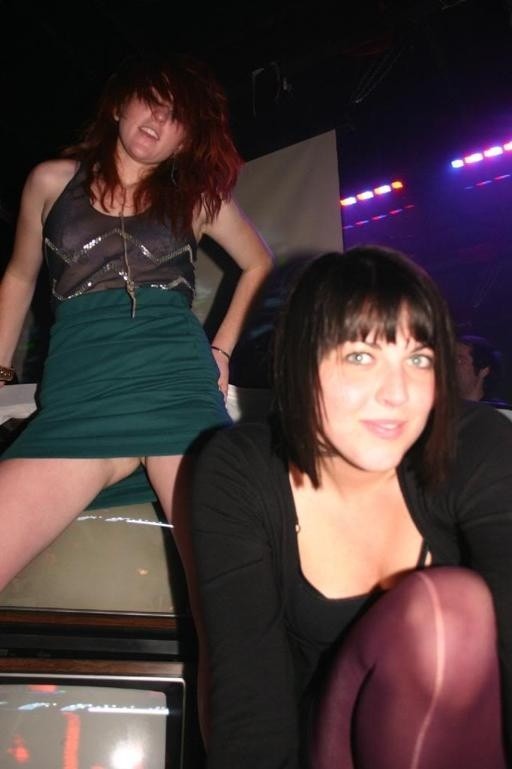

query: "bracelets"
(209, 346), (231, 359)
(1, 366), (15, 381)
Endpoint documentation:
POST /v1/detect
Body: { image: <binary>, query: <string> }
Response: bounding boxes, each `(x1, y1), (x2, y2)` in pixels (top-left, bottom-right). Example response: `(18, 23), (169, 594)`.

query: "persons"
(453, 334), (511, 425)
(0, 57), (275, 594)
(191, 244), (510, 769)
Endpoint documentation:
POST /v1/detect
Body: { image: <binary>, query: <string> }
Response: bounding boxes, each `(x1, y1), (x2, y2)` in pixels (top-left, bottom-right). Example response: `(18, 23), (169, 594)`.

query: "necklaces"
(118, 179), (144, 319)
